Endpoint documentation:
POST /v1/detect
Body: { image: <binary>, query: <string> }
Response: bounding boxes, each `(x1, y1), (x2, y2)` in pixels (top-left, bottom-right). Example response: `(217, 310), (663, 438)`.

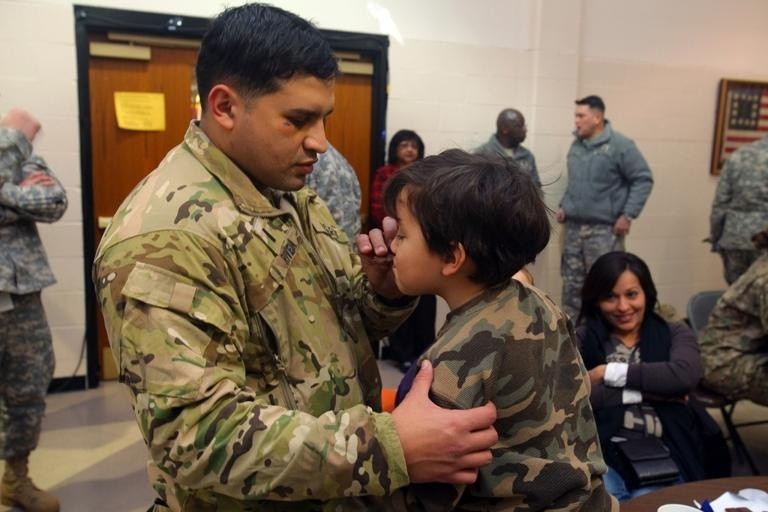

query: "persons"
(387, 149), (623, 512)
(553, 95), (655, 328)
(90, 3), (497, 511)
(304, 142), (363, 254)
(695, 247), (767, 404)
(708, 133), (767, 286)
(1, 106), (82, 510)
(569, 251), (734, 503)
(469, 109), (544, 207)
(366, 129), (436, 366)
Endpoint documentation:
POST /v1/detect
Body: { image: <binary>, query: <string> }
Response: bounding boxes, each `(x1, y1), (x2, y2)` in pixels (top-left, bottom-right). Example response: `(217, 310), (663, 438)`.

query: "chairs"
(687, 289), (768, 477)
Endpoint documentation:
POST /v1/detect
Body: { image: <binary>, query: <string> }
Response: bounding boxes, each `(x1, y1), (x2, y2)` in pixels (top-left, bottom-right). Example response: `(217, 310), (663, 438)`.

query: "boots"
(0, 455), (60, 512)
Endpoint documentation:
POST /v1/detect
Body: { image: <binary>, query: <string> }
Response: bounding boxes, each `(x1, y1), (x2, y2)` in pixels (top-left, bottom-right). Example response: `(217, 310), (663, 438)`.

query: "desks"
(618, 475), (768, 512)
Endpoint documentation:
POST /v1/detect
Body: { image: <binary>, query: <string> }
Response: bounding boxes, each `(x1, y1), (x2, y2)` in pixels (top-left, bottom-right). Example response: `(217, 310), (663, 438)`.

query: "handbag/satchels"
(618, 437), (680, 488)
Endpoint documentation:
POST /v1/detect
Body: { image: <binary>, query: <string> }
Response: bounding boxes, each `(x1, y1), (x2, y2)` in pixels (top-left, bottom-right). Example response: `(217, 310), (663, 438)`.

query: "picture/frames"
(711, 78), (768, 175)
